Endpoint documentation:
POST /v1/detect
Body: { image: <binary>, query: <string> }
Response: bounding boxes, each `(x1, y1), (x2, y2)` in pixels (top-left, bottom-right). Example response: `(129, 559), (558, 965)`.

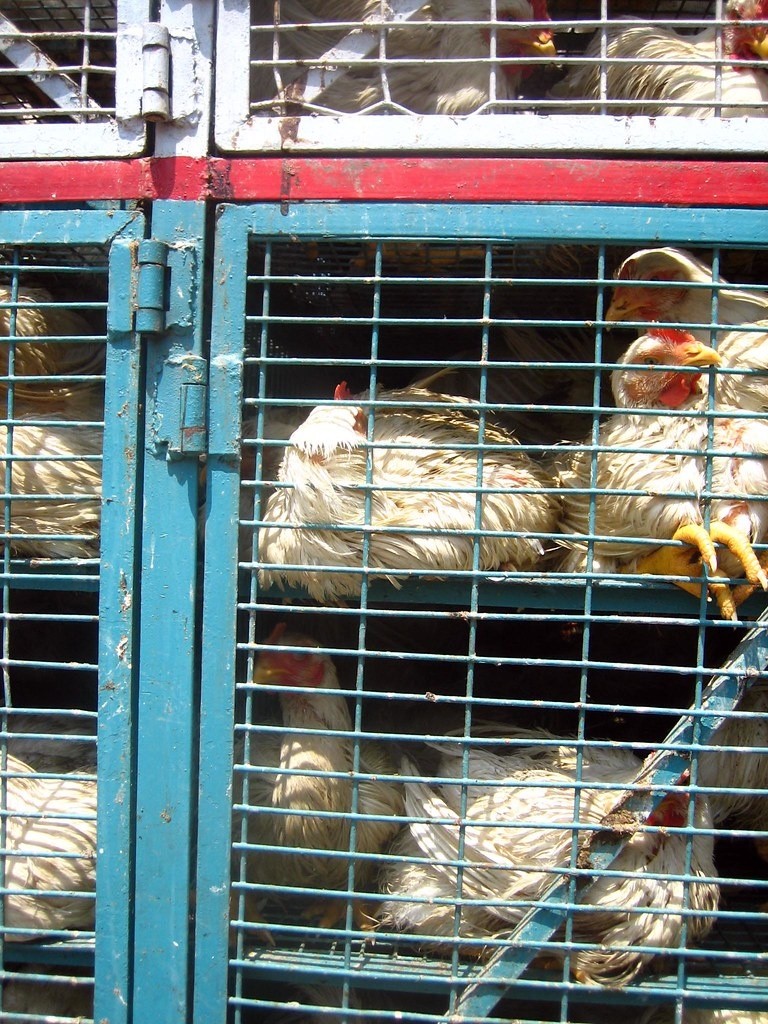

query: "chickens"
(0, 0), (767, 1024)
